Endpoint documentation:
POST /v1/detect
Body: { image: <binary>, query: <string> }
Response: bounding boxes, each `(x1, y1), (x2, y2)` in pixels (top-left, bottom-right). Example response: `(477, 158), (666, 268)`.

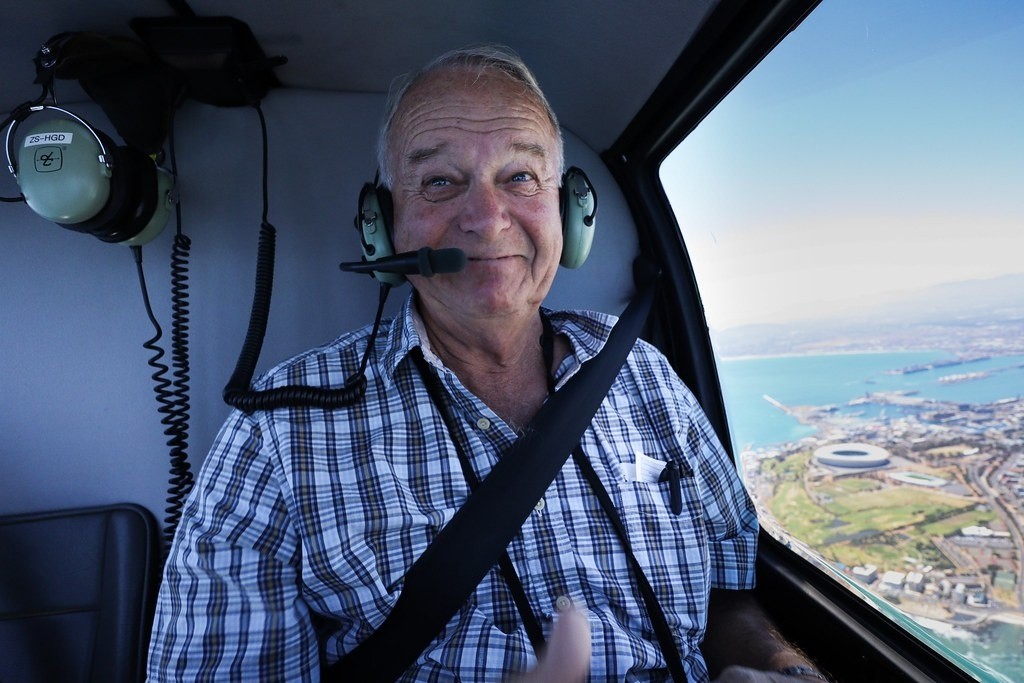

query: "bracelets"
(782, 666), (827, 681)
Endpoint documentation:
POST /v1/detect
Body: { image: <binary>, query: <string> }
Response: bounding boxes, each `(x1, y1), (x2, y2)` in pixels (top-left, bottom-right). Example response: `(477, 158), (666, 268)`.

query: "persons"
(145, 46), (828, 683)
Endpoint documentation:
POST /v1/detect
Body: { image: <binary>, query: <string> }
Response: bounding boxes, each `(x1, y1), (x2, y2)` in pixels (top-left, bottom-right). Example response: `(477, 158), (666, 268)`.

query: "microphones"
(340, 247), (466, 279)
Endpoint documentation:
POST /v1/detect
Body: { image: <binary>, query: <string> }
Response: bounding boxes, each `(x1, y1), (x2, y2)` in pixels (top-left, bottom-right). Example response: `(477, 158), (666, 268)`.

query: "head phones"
(0, 31), (175, 246)
(353, 162), (599, 288)
